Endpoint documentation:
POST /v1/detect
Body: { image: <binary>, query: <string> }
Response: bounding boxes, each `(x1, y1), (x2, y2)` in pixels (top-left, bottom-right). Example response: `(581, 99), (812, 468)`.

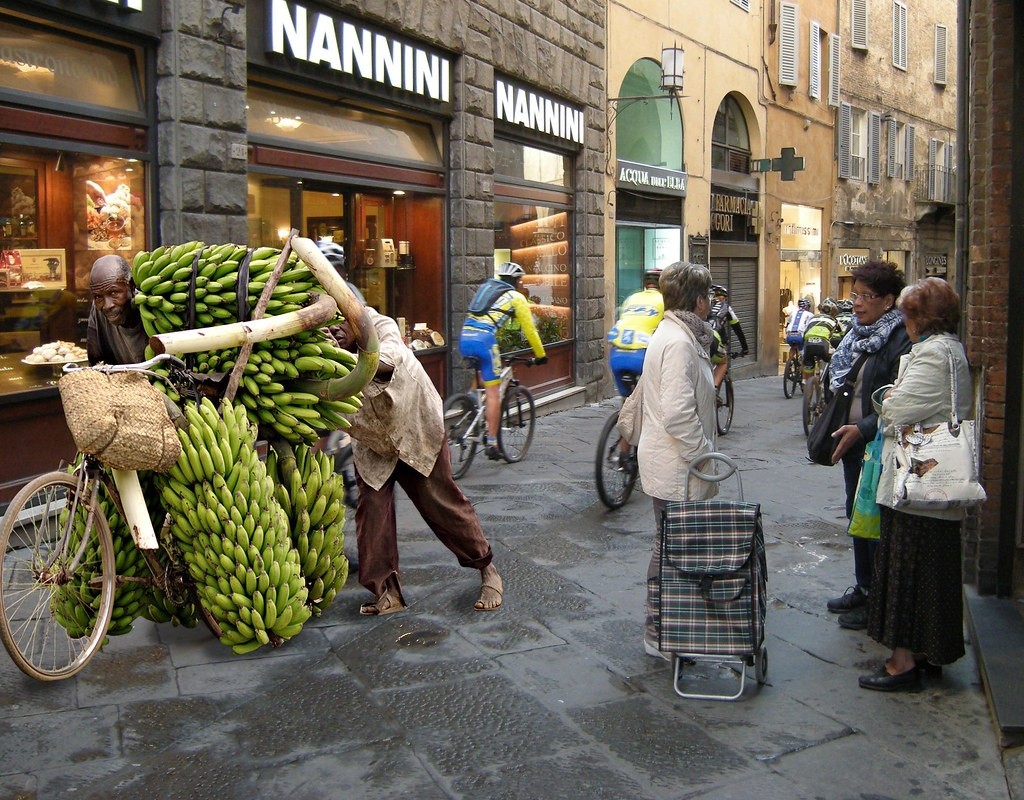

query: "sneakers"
(827, 585), (869, 614)
(838, 606), (868, 630)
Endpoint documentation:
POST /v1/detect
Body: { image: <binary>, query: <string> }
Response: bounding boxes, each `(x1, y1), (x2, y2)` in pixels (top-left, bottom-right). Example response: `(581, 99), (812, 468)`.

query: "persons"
(606, 268), (666, 475)
(638, 262), (720, 660)
(704, 286), (749, 405)
(459, 261), (544, 458)
(38, 290), (80, 351)
(323, 280), (504, 616)
(829, 299), (855, 354)
(786, 298), (814, 375)
(802, 298), (844, 408)
(85, 254), (154, 376)
(857, 277), (974, 693)
(825, 262), (917, 630)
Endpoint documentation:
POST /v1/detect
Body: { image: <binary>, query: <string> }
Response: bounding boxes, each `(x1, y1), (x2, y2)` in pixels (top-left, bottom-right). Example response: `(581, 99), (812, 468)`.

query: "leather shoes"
(915, 657), (943, 683)
(859, 665), (922, 693)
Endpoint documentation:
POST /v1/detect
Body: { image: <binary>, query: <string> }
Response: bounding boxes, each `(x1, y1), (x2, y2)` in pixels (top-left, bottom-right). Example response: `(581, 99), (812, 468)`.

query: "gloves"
(533, 357), (549, 365)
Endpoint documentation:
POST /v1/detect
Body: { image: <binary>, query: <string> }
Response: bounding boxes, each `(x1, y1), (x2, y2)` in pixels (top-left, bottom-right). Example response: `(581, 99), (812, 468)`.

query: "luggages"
(643, 452), (769, 701)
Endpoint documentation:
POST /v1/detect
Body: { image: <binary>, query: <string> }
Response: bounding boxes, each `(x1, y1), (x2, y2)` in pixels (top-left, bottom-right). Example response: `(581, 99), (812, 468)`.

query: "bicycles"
(0, 354), (205, 684)
(708, 349), (748, 435)
(776, 339), (805, 399)
(801, 348), (828, 440)
(439, 355), (541, 479)
(593, 372), (644, 510)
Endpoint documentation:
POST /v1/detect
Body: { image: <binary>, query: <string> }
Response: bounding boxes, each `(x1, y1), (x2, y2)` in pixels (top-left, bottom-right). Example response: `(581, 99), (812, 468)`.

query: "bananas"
(47, 245), (366, 655)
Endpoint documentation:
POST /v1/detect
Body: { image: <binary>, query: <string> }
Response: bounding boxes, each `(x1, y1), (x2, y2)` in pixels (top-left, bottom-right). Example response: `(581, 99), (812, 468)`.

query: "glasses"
(707, 289), (715, 300)
(848, 292), (886, 301)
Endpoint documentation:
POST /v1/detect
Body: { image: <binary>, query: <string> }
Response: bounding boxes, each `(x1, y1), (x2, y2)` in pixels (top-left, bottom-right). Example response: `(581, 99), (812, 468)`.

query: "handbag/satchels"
(892, 339), (987, 511)
(617, 373), (643, 447)
(846, 417), (884, 543)
(58, 363), (182, 473)
(807, 351), (871, 466)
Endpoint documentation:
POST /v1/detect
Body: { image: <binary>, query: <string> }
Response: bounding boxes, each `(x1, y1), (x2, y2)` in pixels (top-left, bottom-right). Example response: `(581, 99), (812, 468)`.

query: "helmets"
(495, 262), (526, 277)
(643, 268), (664, 280)
(798, 299), (811, 307)
(708, 285), (728, 297)
(818, 297), (853, 317)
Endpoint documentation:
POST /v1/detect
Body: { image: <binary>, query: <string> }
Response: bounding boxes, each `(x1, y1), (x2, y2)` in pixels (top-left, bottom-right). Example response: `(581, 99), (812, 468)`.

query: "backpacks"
(466, 278), (516, 329)
(709, 301), (729, 357)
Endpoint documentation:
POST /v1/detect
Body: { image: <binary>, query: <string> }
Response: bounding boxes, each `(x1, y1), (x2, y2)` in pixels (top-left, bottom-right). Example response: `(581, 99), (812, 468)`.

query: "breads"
(26, 341), (88, 364)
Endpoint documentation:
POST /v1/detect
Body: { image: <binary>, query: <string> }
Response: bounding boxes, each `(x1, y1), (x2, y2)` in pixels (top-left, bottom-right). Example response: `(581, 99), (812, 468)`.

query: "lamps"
(606, 38), (686, 175)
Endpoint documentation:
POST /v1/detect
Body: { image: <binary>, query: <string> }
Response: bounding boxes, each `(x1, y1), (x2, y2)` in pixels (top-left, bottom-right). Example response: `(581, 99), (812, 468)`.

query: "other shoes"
(466, 389), (484, 408)
(716, 394), (723, 403)
(485, 446), (504, 459)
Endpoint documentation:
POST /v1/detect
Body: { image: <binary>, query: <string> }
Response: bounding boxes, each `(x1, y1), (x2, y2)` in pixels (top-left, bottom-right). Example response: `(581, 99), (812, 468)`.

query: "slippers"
(473, 584), (504, 612)
(360, 593), (404, 616)
